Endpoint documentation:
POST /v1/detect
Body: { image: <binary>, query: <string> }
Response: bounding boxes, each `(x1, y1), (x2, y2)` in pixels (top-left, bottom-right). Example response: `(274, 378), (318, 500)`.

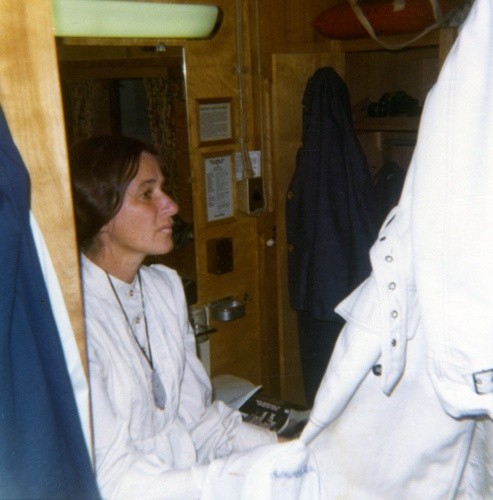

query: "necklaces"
(104, 266), (167, 412)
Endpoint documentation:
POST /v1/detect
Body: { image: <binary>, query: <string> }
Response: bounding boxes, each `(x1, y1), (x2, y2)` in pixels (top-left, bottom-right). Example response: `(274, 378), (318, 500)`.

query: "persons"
(69, 135), (301, 500)
